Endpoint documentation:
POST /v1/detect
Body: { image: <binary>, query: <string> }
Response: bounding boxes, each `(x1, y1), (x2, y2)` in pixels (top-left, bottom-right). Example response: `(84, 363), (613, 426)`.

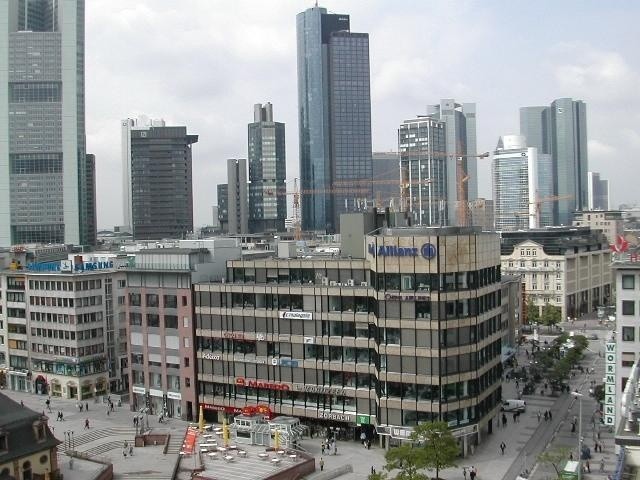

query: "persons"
(19, 394), (168, 459)
(500, 441), (507, 456)
(502, 322), (584, 427)
(470, 470), (476, 480)
(298, 426), (372, 456)
(319, 457), (325, 471)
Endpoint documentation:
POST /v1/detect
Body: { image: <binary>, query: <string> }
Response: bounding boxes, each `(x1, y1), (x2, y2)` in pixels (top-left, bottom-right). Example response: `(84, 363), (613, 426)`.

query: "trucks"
(559, 461), (583, 480)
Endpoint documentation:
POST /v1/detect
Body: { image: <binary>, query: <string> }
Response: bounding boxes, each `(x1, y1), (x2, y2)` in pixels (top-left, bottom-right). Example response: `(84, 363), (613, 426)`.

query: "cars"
(505, 366), (529, 381)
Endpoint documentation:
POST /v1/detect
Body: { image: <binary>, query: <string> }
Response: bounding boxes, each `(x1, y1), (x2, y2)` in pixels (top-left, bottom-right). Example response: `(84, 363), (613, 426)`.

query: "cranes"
(494, 183), (577, 230)
(255, 129), (497, 246)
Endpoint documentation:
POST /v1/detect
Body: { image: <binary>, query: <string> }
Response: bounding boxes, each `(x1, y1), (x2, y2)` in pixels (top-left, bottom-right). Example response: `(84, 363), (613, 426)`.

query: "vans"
(500, 400), (527, 413)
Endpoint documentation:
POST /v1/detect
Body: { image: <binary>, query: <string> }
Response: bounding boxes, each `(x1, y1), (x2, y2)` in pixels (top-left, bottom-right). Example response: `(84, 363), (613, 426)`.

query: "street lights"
(567, 391), (583, 478)
(326, 426), (341, 456)
(528, 360), (539, 387)
(596, 300), (617, 344)
(566, 315), (579, 331)
